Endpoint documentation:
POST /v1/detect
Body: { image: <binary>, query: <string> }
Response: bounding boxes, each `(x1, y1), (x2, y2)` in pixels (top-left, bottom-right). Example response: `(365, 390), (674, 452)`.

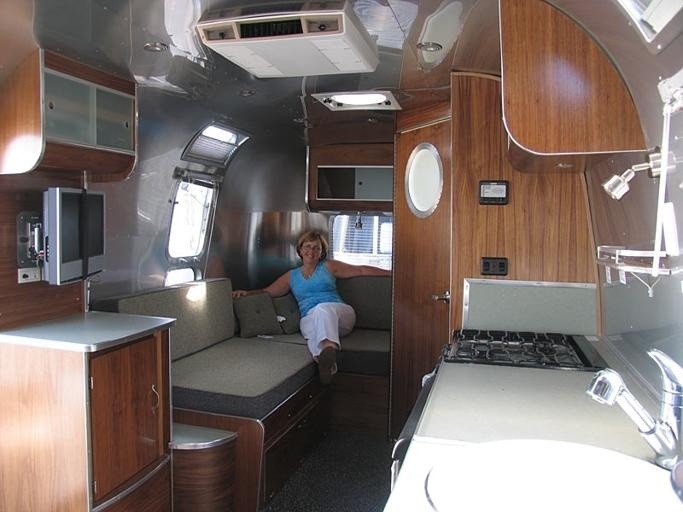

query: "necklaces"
(299, 264), (314, 279)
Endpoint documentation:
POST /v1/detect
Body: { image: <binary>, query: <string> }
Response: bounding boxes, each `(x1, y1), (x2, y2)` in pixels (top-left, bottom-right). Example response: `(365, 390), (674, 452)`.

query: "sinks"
(424, 439), (682, 506)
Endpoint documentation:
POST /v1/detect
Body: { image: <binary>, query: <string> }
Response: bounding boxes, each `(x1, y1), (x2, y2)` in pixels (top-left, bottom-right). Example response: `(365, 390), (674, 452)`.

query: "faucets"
(585, 345), (682, 473)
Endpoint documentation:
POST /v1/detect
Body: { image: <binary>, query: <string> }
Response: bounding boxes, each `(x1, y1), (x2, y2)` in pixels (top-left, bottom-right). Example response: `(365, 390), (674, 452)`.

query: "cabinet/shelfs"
(0, 312), (178, 511)
(0, 46), (138, 183)
(305, 142), (393, 212)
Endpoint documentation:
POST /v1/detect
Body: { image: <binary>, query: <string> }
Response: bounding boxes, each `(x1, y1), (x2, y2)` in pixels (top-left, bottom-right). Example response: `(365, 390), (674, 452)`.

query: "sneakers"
(320, 350), (335, 385)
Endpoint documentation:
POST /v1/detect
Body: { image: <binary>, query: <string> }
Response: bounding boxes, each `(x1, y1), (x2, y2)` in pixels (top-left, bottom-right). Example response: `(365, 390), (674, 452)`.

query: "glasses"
(302, 245), (320, 251)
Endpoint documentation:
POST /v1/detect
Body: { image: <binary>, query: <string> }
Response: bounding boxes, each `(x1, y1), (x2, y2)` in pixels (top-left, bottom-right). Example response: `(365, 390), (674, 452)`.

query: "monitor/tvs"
(42, 187), (105, 286)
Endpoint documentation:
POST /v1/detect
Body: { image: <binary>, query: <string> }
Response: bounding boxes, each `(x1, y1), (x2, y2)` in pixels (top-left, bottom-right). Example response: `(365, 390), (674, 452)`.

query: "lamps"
(602, 152), (677, 198)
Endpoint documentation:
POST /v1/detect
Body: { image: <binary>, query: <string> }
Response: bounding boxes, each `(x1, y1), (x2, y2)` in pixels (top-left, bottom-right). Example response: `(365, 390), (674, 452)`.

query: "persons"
(227, 228), (396, 385)
